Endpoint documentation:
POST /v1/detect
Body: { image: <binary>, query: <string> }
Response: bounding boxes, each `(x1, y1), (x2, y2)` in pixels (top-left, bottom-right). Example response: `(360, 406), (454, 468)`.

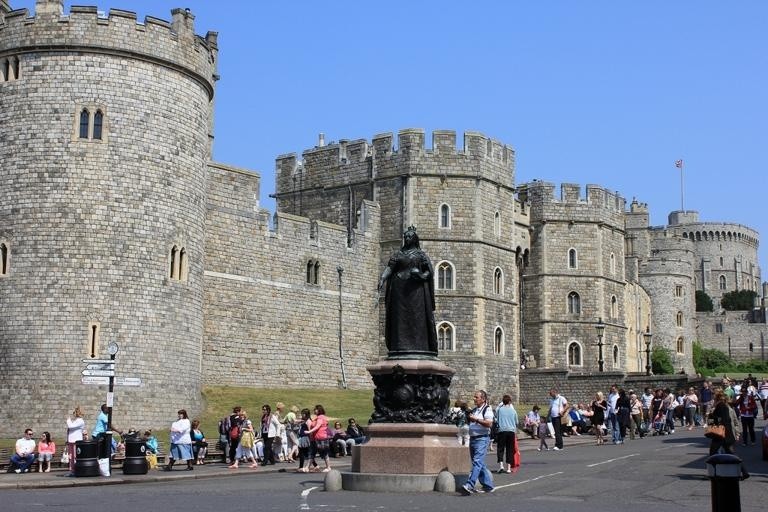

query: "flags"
(675, 160), (683, 167)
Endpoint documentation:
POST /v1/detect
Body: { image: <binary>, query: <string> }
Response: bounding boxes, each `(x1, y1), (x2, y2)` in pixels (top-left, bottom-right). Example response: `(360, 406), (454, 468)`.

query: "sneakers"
(461, 484), (495, 495)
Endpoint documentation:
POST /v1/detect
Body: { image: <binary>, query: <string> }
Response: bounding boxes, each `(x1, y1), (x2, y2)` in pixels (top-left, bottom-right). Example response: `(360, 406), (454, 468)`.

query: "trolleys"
(649, 408), (671, 436)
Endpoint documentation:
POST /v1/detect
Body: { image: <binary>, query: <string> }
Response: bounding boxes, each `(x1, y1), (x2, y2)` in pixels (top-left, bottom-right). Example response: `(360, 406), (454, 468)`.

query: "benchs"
(0, 441), (168, 471)
(206, 438), (224, 465)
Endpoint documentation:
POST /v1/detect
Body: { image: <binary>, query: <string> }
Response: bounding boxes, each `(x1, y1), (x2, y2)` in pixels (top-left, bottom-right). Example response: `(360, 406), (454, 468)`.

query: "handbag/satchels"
(704, 424), (725, 439)
(241, 431), (254, 448)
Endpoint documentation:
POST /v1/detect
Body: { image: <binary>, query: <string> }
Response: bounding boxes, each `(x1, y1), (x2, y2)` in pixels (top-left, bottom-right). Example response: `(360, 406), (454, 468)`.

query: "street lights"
(594, 316), (605, 371)
(642, 325), (651, 375)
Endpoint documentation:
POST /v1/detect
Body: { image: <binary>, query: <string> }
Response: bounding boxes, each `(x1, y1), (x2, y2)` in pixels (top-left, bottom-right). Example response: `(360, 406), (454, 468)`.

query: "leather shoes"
(497, 466), (511, 473)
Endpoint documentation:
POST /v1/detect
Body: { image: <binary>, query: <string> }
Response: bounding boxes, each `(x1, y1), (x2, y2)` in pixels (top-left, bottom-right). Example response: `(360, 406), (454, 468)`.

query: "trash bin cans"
(122, 441), (148, 474)
(74, 441), (99, 478)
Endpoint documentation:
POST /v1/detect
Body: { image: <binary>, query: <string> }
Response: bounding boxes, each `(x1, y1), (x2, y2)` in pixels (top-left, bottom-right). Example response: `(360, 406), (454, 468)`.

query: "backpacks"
(229, 425), (239, 440)
(482, 406), (499, 439)
(217, 416), (231, 435)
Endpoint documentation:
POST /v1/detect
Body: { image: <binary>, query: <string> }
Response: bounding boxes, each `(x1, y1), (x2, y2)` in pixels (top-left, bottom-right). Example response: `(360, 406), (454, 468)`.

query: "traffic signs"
(80, 376), (110, 386)
(81, 358), (115, 364)
(80, 369), (115, 377)
(85, 363), (115, 369)
(113, 375), (143, 386)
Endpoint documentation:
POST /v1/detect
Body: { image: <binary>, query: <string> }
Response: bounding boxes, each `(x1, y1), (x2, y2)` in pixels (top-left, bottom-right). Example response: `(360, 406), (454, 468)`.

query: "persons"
(109, 401), (367, 473)
(607, 382), (710, 443)
(378, 224), (438, 352)
(10, 429), (36, 474)
(91, 404), (123, 440)
(448, 389), (518, 495)
(63, 408), (85, 476)
(707, 377), (768, 447)
(704, 394), (750, 481)
(38, 432), (56, 473)
(523, 389), (607, 450)
